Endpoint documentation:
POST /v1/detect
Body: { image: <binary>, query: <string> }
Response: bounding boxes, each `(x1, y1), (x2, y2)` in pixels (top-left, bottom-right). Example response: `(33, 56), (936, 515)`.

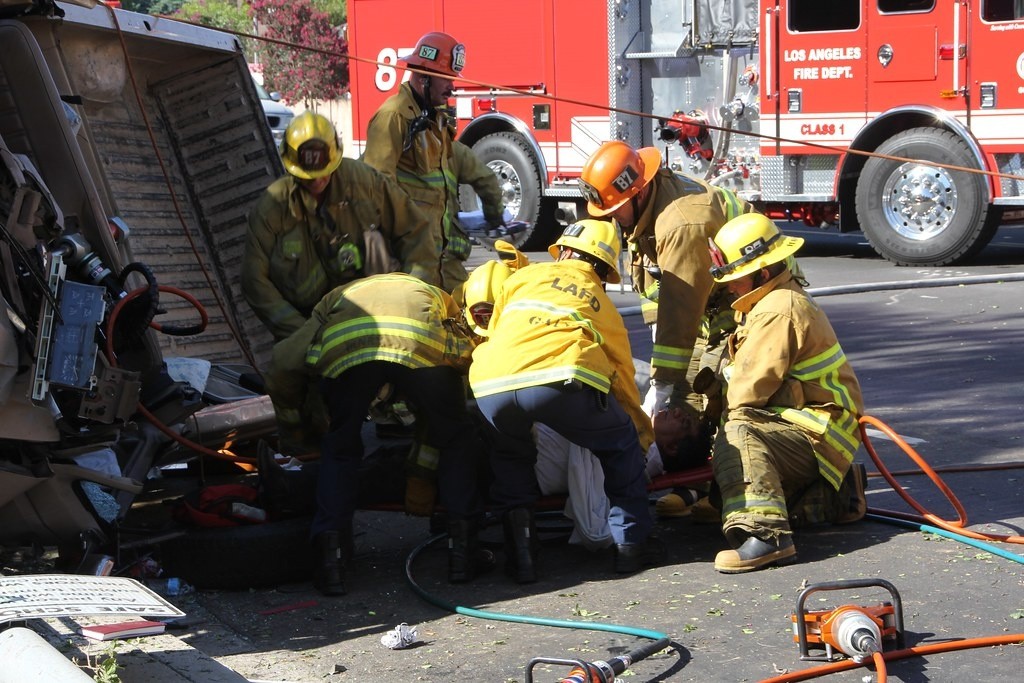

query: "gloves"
(404, 477), (435, 518)
(483, 214), (507, 236)
(643, 441), (664, 482)
(642, 379), (674, 417)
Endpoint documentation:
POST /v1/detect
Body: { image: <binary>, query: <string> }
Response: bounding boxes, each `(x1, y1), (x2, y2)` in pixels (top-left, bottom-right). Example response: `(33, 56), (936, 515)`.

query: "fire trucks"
(344, 1), (1024, 266)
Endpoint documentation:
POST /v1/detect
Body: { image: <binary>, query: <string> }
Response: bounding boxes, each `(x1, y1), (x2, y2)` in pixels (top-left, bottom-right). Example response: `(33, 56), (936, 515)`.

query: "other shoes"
(715, 532), (799, 574)
(832, 463), (868, 526)
(656, 487), (708, 518)
(691, 494), (722, 524)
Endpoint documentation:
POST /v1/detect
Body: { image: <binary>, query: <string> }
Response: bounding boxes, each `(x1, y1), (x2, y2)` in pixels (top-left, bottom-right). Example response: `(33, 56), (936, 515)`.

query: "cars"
(253, 77), (294, 147)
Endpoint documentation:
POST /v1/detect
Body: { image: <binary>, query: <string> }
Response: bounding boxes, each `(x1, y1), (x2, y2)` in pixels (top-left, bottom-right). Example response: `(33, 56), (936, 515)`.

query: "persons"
(364, 33), (466, 289)
(451, 257), (523, 347)
(578, 141), (809, 522)
(240, 110), (446, 340)
(467, 218), (657, 576)
(268, 267), (483, 594)
(710, 212), (868, 574)
(442, 139), (507, 292)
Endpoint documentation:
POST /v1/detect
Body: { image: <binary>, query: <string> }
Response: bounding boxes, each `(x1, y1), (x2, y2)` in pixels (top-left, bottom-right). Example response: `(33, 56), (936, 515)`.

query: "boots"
(614, 542), (644, 573)
(501, 506), (537, 584)
(446, 513), (496, 584)
(312, 530), (348, 597)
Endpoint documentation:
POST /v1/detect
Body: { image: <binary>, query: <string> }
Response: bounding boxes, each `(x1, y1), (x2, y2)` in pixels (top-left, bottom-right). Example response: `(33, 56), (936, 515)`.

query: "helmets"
(400, 32), (466, 78)
(280, 110), (343, 180)
(708, 212), (804, 282)
(548, 219), (622, 284)
(575, 142), (661, 217)
